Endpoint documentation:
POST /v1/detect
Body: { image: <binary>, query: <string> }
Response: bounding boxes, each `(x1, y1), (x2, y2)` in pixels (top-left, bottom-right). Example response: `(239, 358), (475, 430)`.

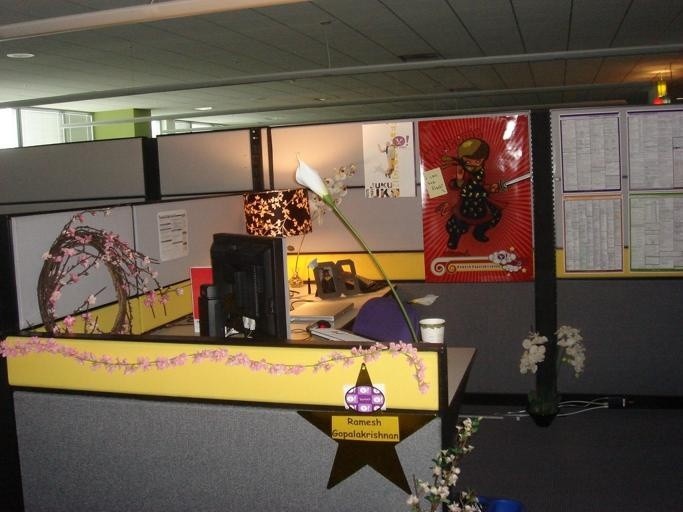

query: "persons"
(321, 268), (336, 294)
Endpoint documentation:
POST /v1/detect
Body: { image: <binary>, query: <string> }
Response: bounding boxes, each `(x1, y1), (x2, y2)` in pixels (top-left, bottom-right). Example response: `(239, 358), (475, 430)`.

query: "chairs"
(351, 294), (421, 345)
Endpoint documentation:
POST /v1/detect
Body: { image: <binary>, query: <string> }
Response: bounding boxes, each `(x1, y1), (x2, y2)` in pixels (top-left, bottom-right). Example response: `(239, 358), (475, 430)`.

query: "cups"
(418, 317), (446, 344)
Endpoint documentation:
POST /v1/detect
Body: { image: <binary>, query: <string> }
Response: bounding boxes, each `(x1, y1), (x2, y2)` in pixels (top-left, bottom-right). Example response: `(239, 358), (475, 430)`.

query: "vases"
(288, 271), (303, 288)
(524, 402), (560, 428)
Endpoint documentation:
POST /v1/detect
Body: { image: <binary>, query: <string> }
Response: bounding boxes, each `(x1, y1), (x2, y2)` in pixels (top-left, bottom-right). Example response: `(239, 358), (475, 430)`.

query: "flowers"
(517, 322), (589, 412)
(2, 336), (430, 396)
(289, 155), (420, 341)
(291, 158), (359, 272)
(32, 206), (187, 338)
(405, 410), (489, 512)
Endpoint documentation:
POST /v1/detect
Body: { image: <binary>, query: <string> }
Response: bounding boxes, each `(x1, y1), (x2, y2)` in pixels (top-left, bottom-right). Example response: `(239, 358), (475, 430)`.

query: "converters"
(608, 397), (626, 409)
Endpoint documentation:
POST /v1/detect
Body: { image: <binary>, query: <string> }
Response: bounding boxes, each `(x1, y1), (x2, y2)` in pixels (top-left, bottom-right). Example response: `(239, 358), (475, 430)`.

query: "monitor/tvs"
(210, 232), (289, 342)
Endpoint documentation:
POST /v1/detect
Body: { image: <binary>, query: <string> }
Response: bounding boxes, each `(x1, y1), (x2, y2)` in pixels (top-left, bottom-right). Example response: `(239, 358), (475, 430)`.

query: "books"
(291, 301), (354, 322)
(311, 327), (376, 343)
(188, 264), (213, 335)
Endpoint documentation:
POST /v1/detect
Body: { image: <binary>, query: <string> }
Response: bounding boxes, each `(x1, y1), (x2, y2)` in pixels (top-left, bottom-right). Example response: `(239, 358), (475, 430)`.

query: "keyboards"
(311, 328), (373, 341)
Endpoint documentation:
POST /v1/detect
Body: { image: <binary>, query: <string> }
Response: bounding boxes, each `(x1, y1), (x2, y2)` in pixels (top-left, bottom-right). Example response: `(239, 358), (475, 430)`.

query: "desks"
(135, 278), (482, 443)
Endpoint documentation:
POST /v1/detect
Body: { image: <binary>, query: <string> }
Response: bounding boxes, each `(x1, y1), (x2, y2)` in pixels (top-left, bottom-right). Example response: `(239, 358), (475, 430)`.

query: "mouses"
(317, 319), (331, 329)
